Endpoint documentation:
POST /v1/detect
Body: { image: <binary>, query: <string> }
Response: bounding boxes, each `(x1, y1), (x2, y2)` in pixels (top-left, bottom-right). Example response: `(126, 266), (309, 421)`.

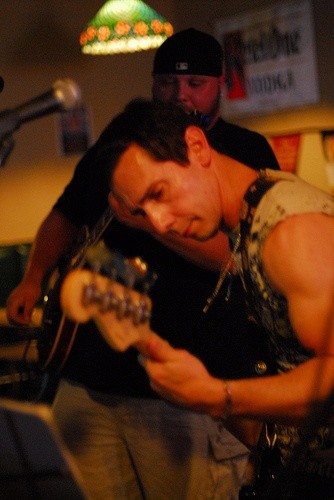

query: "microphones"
(0, 79), (83, 139)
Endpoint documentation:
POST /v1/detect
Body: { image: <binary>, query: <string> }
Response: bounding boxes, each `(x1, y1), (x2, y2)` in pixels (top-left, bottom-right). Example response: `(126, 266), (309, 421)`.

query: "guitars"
(59, 265), (277, 457)
(33, 205), (124, 373)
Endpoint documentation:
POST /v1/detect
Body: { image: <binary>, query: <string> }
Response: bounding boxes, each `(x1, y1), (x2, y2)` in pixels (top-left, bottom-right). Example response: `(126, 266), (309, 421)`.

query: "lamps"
(80, 0), (175, 57)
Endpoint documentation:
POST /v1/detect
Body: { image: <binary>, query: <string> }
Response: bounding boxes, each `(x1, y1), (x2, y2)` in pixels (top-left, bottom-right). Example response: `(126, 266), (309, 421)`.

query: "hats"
(153, 28), (224, 78)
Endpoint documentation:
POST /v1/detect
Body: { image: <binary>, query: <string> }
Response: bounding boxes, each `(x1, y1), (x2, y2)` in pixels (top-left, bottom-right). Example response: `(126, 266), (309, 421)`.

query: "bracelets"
(206, 379), (233, 419)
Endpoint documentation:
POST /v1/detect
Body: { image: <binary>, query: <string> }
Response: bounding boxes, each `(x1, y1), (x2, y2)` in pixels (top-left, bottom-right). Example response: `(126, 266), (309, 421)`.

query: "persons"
(5, 30), (280, 498)
(88, 118), (334, 499)
